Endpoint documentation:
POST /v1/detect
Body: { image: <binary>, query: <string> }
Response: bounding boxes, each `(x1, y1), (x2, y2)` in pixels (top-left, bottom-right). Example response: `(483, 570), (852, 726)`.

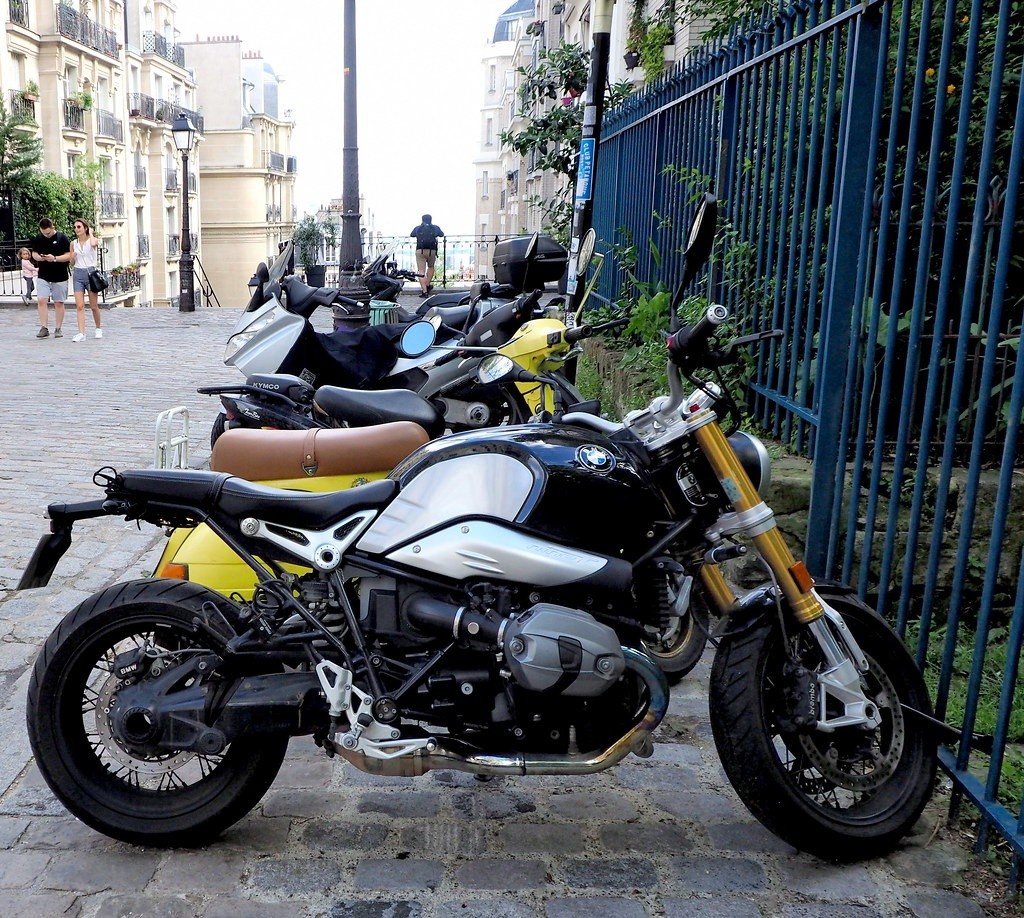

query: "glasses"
(73, 225), (84, 229)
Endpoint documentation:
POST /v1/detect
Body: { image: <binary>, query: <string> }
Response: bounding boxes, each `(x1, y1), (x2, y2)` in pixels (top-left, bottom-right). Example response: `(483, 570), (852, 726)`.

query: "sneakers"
(95, 328), (103, 338)
(28, 298), (37, 303)
(54, 328), (63, 338)
(36, 326), (49, 338)
(23, 296), (29, 306)
(72, 333), (86, 343)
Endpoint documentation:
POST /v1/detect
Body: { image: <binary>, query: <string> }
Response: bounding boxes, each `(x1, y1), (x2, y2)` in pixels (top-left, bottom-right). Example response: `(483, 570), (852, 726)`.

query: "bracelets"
(54, 256), (56, 262)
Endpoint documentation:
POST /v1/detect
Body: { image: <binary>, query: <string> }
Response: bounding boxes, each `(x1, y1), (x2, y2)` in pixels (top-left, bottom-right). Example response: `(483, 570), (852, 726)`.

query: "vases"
(132, 110), (140, 116)
(552, 4), (563, 15)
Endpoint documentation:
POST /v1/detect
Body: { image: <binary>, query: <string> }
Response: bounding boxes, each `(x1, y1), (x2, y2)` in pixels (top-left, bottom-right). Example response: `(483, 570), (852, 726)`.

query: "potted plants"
(111, 264), (140, 276)
(296, 216), (336, 287)
(23, 80), (39, 102)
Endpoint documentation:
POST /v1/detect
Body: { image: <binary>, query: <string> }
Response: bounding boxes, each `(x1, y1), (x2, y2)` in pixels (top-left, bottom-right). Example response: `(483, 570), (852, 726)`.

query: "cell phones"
(40, 254), (46, 257)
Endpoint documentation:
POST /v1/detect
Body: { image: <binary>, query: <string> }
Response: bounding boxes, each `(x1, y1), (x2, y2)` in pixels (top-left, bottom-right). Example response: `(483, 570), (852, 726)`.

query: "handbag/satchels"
(89, 269), (109, 293)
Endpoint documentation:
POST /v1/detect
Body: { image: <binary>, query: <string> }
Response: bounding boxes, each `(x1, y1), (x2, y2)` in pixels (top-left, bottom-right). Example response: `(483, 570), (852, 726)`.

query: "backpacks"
(417, 223), (437, 248)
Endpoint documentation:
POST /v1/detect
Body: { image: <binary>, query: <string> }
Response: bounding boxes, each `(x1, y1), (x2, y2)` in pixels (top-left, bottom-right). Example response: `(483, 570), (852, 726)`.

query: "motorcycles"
(17, 193), (960, 865)
(147, 227), (637, 614)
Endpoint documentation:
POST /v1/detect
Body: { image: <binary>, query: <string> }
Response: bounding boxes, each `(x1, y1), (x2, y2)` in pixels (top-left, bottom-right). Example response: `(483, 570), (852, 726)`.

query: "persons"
(70, 218), (104, 342)
(16, 247), (39, 305)
(32, 218), (71, 338)
(410, 214), (444, 297)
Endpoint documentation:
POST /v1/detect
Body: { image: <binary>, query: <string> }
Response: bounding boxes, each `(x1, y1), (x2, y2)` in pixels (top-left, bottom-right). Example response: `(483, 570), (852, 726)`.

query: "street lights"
(171, 111), (198, 312)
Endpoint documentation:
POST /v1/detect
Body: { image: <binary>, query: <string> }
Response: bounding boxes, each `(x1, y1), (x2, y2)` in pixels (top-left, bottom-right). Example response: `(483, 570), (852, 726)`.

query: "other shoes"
(419, 292), (428, 298)
(426, 284), (434, 293)
